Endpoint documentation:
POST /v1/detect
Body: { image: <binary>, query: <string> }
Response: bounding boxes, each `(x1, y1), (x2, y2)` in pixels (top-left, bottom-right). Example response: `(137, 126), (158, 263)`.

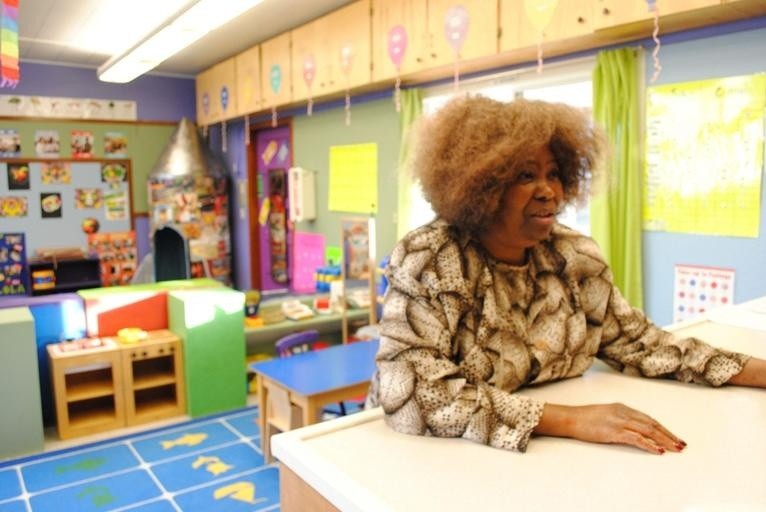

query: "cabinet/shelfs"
(28, 252), (104, 294)
(115, 325), (185, 426)
(45, 337), (127, 438)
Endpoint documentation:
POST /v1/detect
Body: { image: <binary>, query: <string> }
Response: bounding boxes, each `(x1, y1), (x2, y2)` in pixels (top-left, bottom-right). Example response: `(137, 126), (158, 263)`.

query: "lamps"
(93, 0), (262, 85)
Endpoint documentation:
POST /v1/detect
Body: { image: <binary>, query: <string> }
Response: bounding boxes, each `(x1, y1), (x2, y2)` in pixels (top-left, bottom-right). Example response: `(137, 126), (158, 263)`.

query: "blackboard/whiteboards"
(408, 78), (595, 239)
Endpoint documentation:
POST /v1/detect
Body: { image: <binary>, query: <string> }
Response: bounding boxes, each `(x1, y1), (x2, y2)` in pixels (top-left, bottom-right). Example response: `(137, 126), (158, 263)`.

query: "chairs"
(273, 328), (348, 421)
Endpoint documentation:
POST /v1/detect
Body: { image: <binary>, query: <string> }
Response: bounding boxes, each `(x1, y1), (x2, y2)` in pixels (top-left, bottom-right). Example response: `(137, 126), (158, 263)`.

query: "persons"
(365, 93), (766, 456)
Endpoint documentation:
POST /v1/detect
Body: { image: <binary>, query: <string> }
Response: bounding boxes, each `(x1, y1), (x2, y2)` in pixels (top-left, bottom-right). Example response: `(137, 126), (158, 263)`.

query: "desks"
(262, 289), (764, 512)
(245, 290), (380, 398)
(248, 337), (383, 467)
